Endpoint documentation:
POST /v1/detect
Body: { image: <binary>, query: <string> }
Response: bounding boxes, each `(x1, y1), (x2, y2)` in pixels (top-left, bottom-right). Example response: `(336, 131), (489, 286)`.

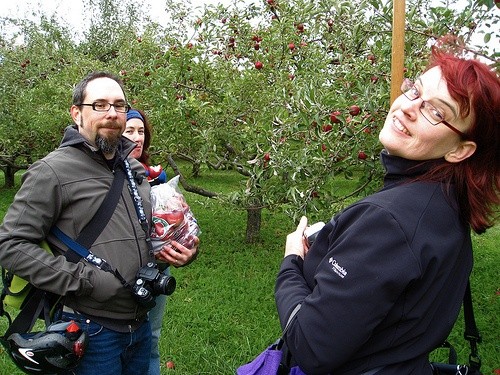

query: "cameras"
(131, 262), (176, 308)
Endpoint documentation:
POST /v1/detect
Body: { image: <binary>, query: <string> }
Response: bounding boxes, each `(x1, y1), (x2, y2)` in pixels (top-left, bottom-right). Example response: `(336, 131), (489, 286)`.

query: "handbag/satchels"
(429, 341), (483, 375)
(236, 304), (305, 375)
(1, 241), (64, 319)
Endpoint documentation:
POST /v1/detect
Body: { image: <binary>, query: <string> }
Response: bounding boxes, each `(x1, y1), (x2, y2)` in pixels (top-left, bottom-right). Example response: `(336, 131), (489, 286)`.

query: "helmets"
(0, 320), (89, 375)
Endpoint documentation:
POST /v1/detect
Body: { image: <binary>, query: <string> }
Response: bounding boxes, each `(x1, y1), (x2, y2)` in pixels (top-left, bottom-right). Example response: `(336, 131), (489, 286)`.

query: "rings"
(174, 260), (178, 264)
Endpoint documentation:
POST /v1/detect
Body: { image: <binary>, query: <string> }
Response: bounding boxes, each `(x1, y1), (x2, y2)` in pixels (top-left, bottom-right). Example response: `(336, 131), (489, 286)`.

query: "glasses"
(75, 102), (131, 113)
(399, 77), (468, 141)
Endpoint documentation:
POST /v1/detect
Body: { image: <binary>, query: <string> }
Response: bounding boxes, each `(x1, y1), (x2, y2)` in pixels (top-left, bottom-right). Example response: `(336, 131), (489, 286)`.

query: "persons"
(121, 108), (167, 375)
(0, 72), (199, 375)
(275, 38), (500, 375)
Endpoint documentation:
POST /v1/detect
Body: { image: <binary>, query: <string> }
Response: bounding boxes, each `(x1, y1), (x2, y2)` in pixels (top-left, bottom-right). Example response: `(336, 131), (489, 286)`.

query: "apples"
(152, 198), (199, 260)
(16, 0), (477, 199)
(494, 369), (500, 375)
(166, 361), (174, 370)
